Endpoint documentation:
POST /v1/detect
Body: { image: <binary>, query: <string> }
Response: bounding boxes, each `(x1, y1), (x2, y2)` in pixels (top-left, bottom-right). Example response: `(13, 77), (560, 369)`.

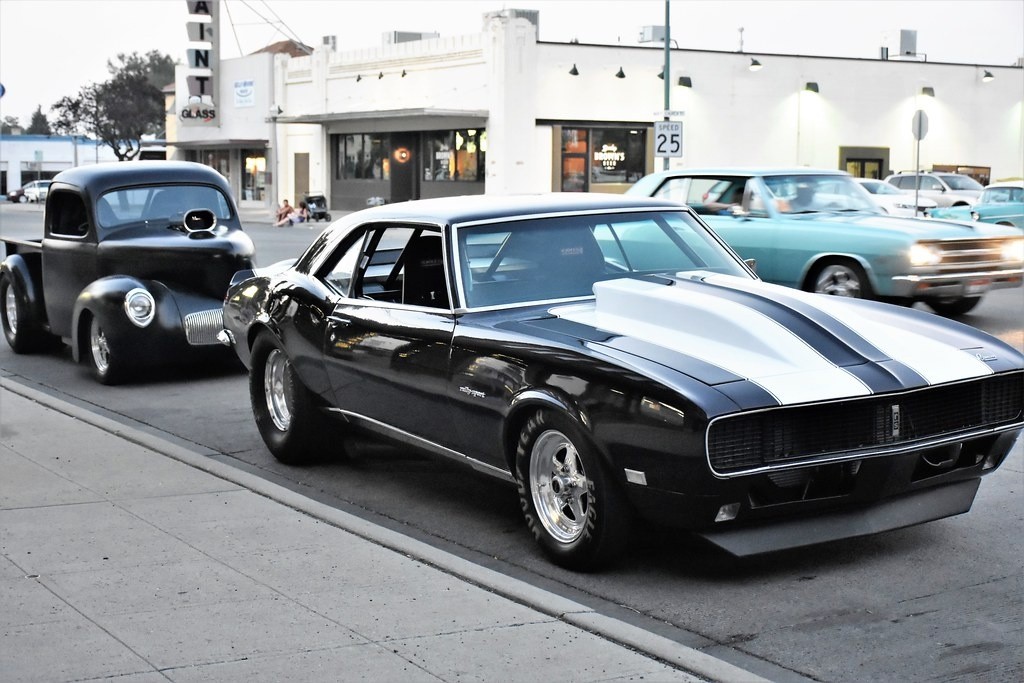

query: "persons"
(273, 202), (308, 227)
(784, 178), (838, 211)
(707, 189), (756, 215)
(275, 200), (294, 226)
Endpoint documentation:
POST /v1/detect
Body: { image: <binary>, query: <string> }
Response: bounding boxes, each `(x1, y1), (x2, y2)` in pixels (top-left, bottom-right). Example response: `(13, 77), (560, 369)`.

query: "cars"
(0, 159), (262, 386)
(466, 165), (1023, 320)
(220, 189), (1024, 575)
(7, 179), (51, 204)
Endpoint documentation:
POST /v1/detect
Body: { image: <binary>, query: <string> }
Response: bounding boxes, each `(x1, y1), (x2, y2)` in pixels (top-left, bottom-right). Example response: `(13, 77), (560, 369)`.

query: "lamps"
(805, 82), (820, 92)
(377, 70), (384, 80)
(566, 61), (580, 76)
(678, 77), (693, 90)
(399, 68), (407, 77)
(354, 71), (363, 84)
(614, 66), (625, 79)
(744, 53), (763, 72)
(270, 104), (284, 120)
(657, 66), (667, 80)
(976, 65), (994, 83)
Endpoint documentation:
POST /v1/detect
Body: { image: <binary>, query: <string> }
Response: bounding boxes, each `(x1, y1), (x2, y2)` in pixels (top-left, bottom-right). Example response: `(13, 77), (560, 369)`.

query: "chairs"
(534, 223), (608, 300)
(142, 190), (188, 220)
(400, 236), (475, 305)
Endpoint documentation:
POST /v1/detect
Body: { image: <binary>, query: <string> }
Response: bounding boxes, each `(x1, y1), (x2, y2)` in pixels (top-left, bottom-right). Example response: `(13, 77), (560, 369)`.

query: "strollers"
(303, 191), (331, 223)
